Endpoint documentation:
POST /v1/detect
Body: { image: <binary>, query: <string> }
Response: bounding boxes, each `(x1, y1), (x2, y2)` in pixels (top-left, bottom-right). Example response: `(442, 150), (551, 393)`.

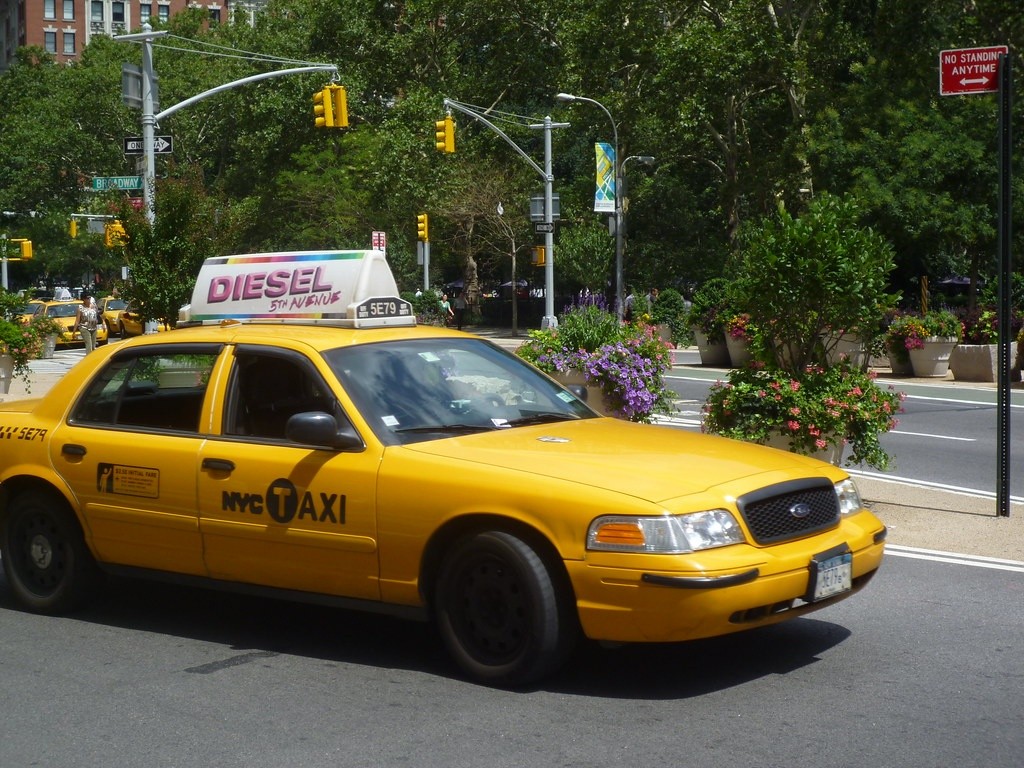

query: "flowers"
(403, 290), (453, 331)
(700, 347), (909, 474)
(968, 308), (1022, 346)
(511, 303), (682, 427)
(878, 311), (967, 353)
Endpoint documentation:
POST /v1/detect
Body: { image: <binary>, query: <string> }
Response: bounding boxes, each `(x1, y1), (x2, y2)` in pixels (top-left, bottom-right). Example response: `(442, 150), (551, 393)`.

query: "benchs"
(113, 382), (200, 431)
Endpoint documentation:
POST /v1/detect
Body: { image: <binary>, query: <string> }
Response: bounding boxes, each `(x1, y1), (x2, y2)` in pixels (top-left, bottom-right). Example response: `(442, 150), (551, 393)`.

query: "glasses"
(87, 296), (91, 300)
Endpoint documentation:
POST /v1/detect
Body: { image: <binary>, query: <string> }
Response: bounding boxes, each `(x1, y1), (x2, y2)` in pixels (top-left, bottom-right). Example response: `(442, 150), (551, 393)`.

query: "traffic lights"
(529, 247), (545, 265)
(435, 118), (455, 153)
(415, 214), (429, 243)
(312, 89), (334, 128)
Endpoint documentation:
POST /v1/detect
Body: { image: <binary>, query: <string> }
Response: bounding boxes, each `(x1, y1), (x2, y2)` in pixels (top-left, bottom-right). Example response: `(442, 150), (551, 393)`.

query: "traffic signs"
(123, 135), (174, 155)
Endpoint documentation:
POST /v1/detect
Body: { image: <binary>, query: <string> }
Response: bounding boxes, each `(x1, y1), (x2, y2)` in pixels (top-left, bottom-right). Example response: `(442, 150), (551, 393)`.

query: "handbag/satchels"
(96, 312), (103, 324)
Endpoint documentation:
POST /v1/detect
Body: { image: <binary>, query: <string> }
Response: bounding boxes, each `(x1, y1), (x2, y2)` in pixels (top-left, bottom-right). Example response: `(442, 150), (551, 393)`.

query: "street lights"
(556, 92), (656, 324)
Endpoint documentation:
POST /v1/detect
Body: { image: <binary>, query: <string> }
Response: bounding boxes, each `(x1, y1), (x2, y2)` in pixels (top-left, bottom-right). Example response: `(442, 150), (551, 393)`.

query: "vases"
(950, 341), (1017, 383)
(546, 365), (631, 425)
(419, 321), (442, 327)
(745, 426), (846, 468)
(882, 335), (958, 379)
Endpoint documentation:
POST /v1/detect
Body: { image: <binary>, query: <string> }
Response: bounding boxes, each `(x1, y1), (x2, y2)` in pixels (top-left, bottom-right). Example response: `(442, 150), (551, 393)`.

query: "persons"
(415, 288), (423, 298)
(624, 287), (639, 321)
(72, 293), (97, 357)
(438, 288), (444, 296)
(451, 292), (469, 331)
(438, 295), (454, 316)
(645, 287), (659, 316)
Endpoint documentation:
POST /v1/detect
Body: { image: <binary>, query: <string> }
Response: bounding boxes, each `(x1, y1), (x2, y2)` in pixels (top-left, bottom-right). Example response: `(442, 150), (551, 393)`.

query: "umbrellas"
(448, 278), (464, 288)
(501, 279), (528, 288)
(937, 274), (981, 286)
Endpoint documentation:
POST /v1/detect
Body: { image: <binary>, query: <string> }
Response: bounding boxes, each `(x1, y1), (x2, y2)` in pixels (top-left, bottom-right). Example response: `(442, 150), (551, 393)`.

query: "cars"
(0, 249), (887, 692)
(0, 284), (173, 357)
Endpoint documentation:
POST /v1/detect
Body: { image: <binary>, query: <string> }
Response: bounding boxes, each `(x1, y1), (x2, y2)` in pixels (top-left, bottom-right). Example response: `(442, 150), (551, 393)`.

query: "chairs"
(239, 359), (297, 438)
